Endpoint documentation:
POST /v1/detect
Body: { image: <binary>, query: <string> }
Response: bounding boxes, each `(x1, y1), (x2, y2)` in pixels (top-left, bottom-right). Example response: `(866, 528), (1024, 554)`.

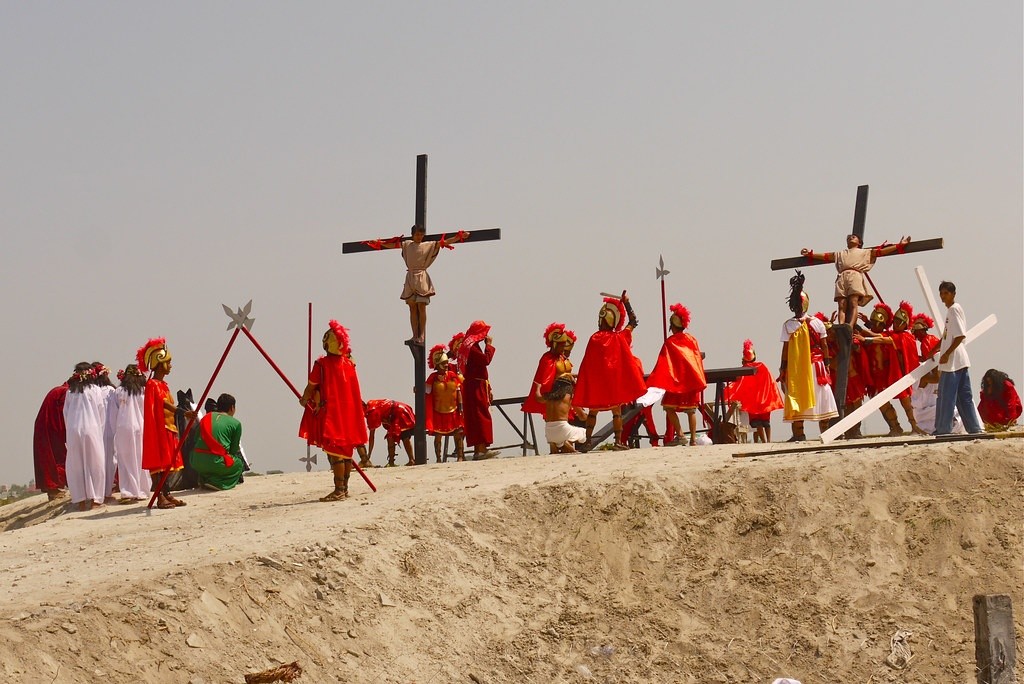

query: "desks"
(439, 366), (756, 457)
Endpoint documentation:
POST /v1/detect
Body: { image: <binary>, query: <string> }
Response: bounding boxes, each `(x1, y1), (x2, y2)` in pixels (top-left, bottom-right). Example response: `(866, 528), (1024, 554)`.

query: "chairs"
(703, 399), (759, 443)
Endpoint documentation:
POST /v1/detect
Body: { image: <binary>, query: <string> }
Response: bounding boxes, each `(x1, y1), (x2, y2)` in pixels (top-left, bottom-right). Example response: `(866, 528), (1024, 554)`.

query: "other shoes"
(889, 429), (903, 435)
(787, 436), (806, 442)
(204, 482), (222, 491)
(121, 497), (136, 505)
(668, 436), (687, 445)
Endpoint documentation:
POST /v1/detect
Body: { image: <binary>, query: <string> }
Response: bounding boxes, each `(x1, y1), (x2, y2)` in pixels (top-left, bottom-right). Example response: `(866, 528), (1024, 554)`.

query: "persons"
(33, 339), (249, 512)
(300, 320), (368, 501)
(520, 270), (1023, 457)
(425, 320), (500, 462)
(801, 235), (911, 332)
(368, 224), (468, 347)
(359, 399), (415, 467)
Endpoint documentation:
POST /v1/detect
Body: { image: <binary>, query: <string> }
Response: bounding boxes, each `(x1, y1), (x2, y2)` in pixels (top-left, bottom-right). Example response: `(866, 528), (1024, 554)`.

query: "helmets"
(742, 340), (755, 361)
(322, 321), (349, 356)
(427, 345), (449, 370)
(564, 330), (576, 352)
(668, 305), (688, 329)
(448, 332), (467, 360)
(787, 273), (808, 315)
(598, 298), (627, 333)
(544, 323), (568, 349)
(137, 339), (172, 373)
(817, 300), (933, 337)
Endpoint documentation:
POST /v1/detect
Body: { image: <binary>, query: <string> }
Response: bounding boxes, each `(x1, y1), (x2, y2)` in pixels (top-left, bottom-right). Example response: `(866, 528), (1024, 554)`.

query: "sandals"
(157, 499), (175, 508)
(167, 494), (187, 506)
(320, 491), (347, 502)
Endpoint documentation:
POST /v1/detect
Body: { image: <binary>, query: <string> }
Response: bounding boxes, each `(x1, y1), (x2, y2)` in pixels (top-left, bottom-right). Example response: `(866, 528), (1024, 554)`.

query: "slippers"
(478, 449), (500, 460)
